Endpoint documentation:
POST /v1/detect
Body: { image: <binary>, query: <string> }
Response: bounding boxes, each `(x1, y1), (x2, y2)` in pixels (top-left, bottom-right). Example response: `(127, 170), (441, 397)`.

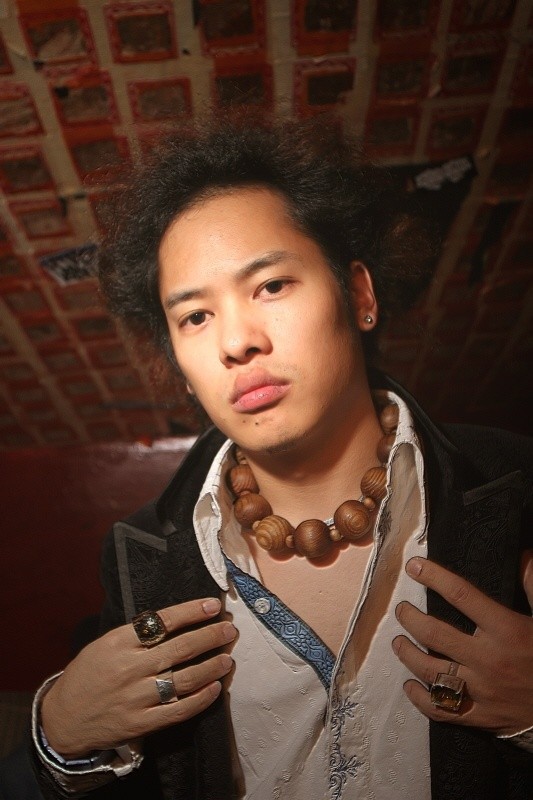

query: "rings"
(429, 662), (465, 710)
(131, 610), (169, 648)
(156, 670), (178, 704)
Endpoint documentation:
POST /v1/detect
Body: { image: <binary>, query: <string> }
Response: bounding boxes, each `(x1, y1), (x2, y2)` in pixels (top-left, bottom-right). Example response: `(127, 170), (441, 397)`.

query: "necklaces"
(224, 400), (401, 558)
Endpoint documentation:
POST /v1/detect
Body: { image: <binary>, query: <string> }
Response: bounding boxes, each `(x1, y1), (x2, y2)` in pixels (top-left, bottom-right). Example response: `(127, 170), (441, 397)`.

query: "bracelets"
(35, 723), (117, 772)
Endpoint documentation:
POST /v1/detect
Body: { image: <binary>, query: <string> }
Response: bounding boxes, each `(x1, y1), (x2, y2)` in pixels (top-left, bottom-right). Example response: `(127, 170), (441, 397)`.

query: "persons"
(23, 104), (533, 800)
